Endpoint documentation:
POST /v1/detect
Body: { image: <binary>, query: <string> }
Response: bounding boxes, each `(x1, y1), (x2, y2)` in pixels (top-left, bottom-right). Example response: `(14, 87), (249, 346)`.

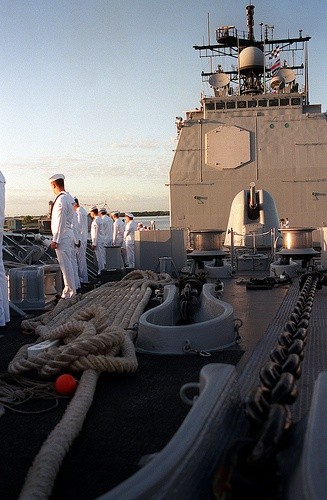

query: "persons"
(48, 173), (151, 299)
(0, 171), (10, 327)
(280, 218), (289, 229)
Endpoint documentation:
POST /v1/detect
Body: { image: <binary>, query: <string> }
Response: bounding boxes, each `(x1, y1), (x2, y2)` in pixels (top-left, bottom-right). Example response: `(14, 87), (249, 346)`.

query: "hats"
(71, 196), (77, 203)
(98, 207), (107, 214)
(125, 212), (134, 218)
(110, 211), (120, 215)
(49, 174), (66, 183)
(89, 207), (98, 211)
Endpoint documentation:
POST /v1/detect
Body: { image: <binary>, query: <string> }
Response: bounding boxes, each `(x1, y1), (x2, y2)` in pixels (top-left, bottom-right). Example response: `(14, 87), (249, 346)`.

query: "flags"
(270, 58), (281, 76)
(269, 45), (279, 60)
